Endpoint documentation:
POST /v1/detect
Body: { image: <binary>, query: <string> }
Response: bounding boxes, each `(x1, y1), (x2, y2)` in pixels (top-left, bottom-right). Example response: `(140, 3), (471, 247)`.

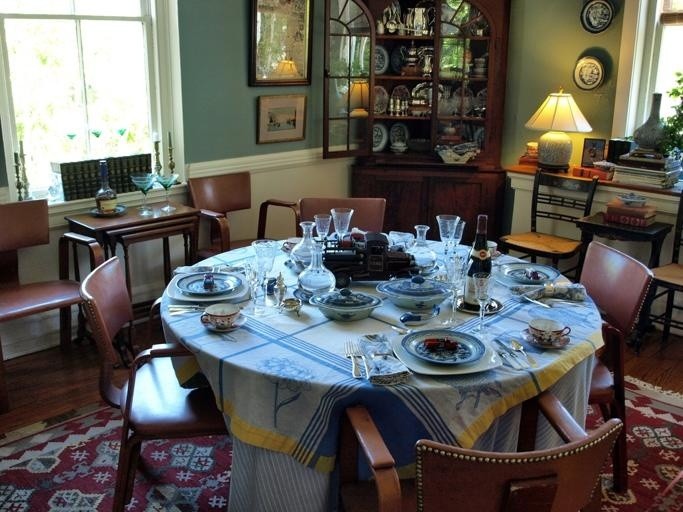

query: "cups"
(527, 141), (537, 158)
(472, 273), (495, 333)
(529, 319), (571, 345)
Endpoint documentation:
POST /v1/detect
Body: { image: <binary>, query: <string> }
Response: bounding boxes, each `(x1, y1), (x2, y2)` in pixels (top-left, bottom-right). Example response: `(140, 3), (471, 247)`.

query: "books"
(607, 198), (657, 218)
(572, 166), (613, 180)
(603, 214), (657, 227)
(612, 155), (683, 189)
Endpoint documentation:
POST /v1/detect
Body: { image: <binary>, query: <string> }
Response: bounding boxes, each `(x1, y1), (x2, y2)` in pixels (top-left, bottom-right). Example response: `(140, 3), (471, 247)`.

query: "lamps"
(524, 89), (592, 173)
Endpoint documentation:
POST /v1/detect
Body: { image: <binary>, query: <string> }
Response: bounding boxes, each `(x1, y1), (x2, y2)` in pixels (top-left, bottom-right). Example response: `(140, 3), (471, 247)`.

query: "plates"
(167, 274), (249, 300)
(177, 272), (242, 295)
(201, 314), (246, 332)
(375, 45), (388, 76)
(453, 294), (504, 314)
(402, 330), (486, 365)
(372, 124), (387, 152)
(374, 86), (388, 115)
(519, 329), (570, 350)
(390, 123), (409, 145)
(89, 207), (125, 218)
(393, 334), (503, 374)
(503, 264), (560, 284)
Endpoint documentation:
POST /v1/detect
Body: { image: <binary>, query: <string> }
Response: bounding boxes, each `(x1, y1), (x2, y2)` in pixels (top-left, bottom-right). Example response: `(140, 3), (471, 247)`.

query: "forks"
(344, 341), (362, 379)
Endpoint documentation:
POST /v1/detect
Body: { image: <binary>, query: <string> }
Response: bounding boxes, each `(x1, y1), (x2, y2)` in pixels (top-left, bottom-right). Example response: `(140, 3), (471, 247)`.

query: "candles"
(14, 153), (20, 176)
(155, 141), (160, 152)
(19, 141), (25, 167)
(168, 132), (172, 148)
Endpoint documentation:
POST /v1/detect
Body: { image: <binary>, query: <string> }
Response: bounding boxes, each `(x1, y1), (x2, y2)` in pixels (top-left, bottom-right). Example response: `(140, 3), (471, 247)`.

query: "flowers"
(633, 93), (665, 149)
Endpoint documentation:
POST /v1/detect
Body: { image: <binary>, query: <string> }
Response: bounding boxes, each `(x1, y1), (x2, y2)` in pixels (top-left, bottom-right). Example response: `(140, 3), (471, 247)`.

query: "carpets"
(0, 370), (683, 512)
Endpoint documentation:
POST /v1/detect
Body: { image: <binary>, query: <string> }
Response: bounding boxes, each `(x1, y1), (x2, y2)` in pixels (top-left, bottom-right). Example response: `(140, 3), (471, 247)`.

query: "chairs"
(0, 199), (102, 415)
(337, 389), (624, 511)
(498, 168), (597, 284)
(299, 198), (386, 239)
(187, 171), (301, 264)
(78, 256), (229, 512)
(578, 241), (656, 493)
(633, 190), (683, 342)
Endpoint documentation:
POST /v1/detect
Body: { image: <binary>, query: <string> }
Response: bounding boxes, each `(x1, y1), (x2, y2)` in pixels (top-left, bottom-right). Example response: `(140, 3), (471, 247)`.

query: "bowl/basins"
(472, 241), (498, 256)
(309, 289), (383, 322)
(376, 275), (453, 310)
(200, 304), (241, 329)
(474, 58), (488, 77)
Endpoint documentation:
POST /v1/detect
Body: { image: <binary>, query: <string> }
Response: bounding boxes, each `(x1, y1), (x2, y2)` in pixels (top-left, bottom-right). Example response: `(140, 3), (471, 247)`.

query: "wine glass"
(436, 215), (460, 243)
(252, 240), (277, 272)
(131, 174), (156, 216)
(314, 214), (331, 236)
(156, 173), (178, 213)
(330, 208), (354, 232)
(448, 221), (466, 242)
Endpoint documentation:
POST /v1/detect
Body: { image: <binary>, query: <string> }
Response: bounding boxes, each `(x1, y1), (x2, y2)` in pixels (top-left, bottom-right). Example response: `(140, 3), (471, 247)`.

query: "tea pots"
(399, 40), (424, 76)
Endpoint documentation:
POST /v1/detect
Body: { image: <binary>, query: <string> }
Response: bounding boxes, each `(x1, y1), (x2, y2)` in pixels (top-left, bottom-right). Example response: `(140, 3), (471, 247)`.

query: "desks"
(575, 211), (673, 348)
(64, 201), (201, 368)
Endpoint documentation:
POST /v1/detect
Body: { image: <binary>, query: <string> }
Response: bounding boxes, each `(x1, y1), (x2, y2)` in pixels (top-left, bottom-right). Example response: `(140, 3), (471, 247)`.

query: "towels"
(507, 284), (576, 302)
(357, 328), (413, 385)
(389, 231), (416, 250)
(174, 265), (246, 274)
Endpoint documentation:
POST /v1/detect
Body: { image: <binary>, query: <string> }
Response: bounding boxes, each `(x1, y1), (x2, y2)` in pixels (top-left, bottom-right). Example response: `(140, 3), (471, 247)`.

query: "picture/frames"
(256, 94), (307, 144)
(580, 138), (605, 167)
(248, 0), (315, 86)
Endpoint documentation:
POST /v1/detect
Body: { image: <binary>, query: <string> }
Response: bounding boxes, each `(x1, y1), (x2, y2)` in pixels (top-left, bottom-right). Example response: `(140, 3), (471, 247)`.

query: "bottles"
(405, 225), (437, 272)
(299, 246), (336, 298)
(96, 160), (118, 213)
(289, 221), (319, 273)
(464, 215), (492, 312)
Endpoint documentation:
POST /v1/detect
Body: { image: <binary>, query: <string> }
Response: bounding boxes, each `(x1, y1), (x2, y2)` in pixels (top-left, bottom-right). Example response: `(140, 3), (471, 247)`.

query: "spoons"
(511, 339), (536, 367)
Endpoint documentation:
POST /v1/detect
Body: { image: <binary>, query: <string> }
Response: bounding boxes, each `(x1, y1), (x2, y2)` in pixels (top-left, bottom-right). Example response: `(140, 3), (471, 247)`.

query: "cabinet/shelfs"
(350, 168), (427, 240)
(427, 171), (507, 255)
(323, 0), (510, 170)
